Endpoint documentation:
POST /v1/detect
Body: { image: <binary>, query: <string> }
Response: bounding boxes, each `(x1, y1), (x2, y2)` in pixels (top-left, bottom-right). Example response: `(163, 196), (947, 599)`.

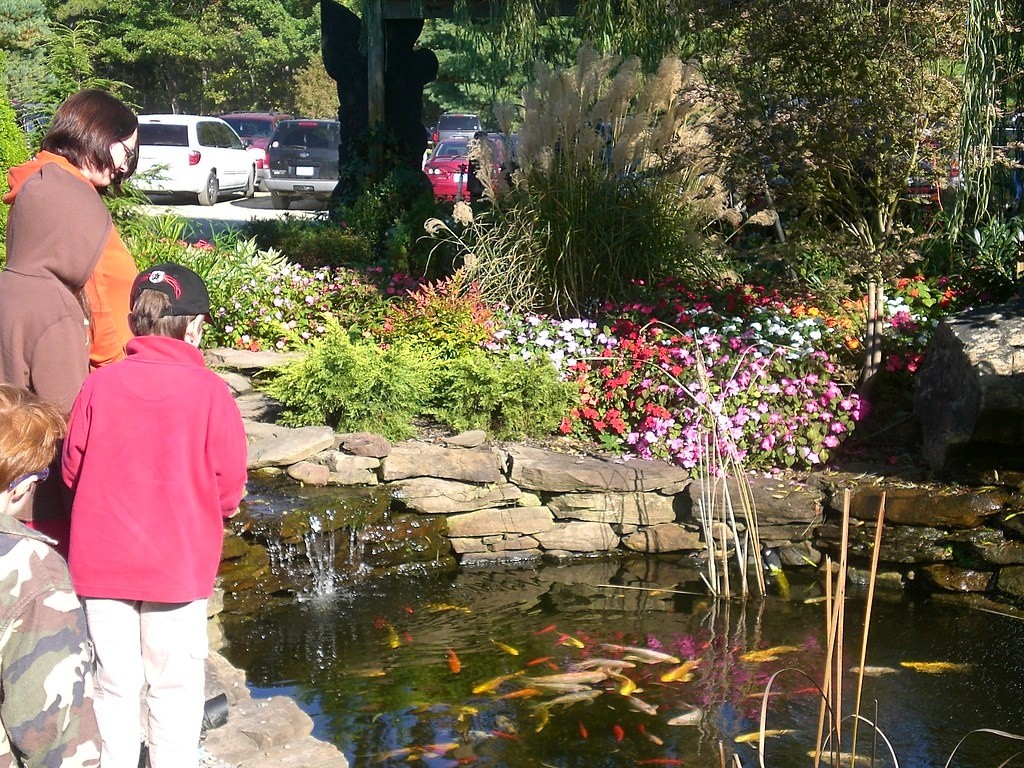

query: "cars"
(117, 113), (258, 206)
(420, 111), (520, 207)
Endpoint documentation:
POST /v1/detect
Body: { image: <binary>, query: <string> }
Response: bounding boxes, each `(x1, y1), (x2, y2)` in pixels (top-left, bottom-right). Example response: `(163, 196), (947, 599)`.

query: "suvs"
(217, 109), (295, 194)
(263, 117), (344, 212)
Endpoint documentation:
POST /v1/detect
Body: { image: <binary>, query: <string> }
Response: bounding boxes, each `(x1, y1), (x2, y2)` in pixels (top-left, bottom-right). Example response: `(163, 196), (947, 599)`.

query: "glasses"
(10, 466), (49, 491)
(115, 138), (138, 170)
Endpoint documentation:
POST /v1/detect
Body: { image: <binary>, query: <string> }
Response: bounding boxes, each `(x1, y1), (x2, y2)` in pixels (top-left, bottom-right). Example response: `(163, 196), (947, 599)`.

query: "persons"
(0, 163), (113, 422)
(2, 88), (144, 370)
(0, 380), (104, 767)
(61, 262), (248, 766)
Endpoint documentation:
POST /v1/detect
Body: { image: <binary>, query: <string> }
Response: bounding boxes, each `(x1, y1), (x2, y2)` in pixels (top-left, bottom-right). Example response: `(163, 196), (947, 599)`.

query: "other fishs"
(368, 598), (477, 649)
(471, 582), (705, 768)
(729, 562), (974, 768)
(330, 650), (496, 768)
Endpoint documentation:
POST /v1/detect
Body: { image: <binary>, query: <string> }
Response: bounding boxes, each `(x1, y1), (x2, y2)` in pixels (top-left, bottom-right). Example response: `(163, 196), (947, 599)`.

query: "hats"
(129, 261), (217, 328)
